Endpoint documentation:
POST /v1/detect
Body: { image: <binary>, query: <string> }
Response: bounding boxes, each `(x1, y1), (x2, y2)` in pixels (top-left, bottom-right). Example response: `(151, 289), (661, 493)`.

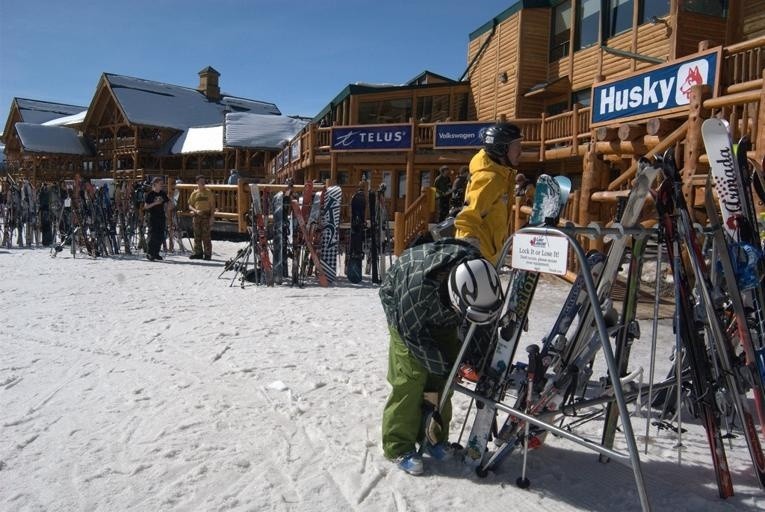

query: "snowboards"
(249, 179), (386, 288)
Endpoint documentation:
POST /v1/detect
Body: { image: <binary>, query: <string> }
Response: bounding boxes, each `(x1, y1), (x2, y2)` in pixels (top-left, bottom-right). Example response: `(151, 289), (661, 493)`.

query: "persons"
(228, 169), (242, 185)
(436, 166), (452, 220)
(143, 178), (175, 261)
(514, 173), (531, 196)
(380, 237), (495, 475)
(189, 175), (217, 260)
(452, 167), (469, 215)
(463, 122), (529, 376)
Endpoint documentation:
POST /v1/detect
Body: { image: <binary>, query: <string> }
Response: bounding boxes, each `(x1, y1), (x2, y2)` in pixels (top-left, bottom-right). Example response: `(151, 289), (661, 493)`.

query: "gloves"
(459, 361), (480, 382)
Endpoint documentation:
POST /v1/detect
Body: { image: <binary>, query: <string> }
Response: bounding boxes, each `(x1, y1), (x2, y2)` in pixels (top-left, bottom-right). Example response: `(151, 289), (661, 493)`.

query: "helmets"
(483, 122), (524, 166)
(447, 254), (505, 327)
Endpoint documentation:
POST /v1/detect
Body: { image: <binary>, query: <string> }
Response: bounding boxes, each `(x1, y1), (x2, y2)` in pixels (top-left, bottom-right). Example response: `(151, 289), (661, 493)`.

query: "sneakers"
(423, 442), (458, 462)
(190, 253), (211, 260)
(146, 253), (163, 261)
(388, 448), (424, 477)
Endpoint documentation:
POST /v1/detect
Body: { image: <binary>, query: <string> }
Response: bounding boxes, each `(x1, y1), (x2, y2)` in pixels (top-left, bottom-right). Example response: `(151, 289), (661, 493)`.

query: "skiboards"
(460, 118), (761, 500)
